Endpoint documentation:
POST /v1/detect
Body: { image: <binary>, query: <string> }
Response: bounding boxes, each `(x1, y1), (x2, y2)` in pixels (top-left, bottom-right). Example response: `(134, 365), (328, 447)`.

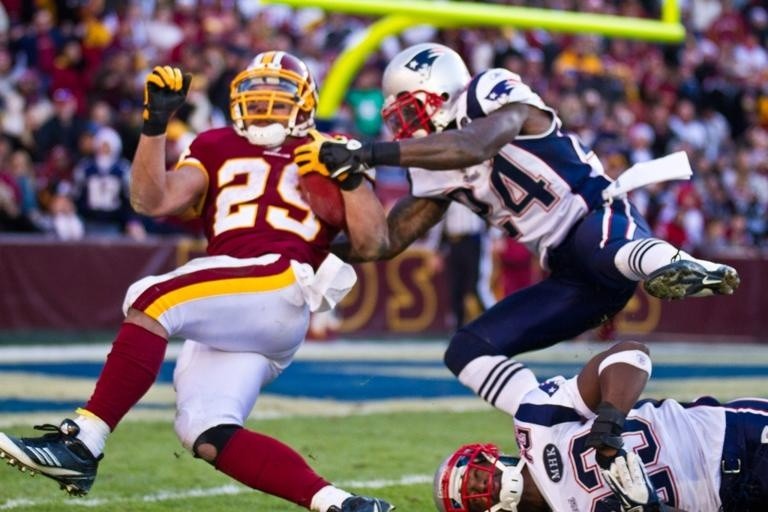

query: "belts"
(720, 413), (741, 501)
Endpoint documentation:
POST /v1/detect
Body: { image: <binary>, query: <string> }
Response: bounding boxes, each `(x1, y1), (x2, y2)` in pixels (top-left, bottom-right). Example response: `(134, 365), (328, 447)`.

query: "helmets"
(433, 443), (526, 512)
(230, 51), (318, 148)
(381, 43), (472, 141)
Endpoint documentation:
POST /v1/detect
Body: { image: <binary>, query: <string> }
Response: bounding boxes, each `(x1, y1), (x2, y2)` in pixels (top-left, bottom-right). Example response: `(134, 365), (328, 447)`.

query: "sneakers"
(0, 418), (104, 496)
(643, 259), (740, 300)
(326, 493), (395, 512)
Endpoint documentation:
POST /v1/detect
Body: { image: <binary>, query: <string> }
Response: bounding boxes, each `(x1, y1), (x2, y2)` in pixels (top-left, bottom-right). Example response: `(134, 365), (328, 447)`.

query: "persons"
(433, 340), (768, 512)
(0, 50), (397, 512)
(0, 0), (768, 242)
(295, 41), (741, 417)
(447, 197), (499, 335)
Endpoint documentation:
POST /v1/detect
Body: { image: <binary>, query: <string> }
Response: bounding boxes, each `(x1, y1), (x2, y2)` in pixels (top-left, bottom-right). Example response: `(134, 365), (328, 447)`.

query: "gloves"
(595, 449), (664, 512)
(293, 127), (400, 191)
(141, 66), (193, 136)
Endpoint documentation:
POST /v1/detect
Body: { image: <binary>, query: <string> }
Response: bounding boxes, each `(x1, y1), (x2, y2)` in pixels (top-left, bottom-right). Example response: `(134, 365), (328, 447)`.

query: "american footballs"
(298, 135), (346, 228)
